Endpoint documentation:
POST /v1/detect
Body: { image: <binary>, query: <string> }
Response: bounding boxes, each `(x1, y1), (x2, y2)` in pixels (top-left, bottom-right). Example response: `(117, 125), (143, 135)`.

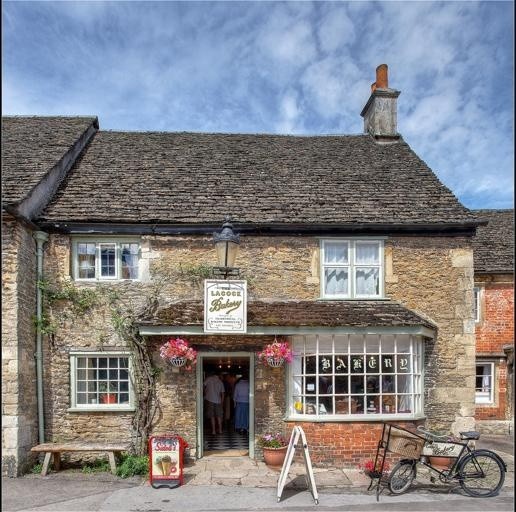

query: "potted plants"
(98, 381), (118, 403)
(427, 443), (459, 466)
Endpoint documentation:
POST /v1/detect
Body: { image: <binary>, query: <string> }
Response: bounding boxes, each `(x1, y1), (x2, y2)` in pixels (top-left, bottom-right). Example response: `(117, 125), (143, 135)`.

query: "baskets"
(383, 425), (425, 460)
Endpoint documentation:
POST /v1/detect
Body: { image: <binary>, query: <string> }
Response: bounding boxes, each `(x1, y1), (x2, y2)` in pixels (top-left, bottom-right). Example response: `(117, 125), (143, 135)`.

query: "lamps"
(209, 212), (244, 277)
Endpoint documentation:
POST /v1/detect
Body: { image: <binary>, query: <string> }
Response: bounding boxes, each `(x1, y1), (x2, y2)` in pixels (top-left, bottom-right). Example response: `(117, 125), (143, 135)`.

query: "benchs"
(30, 440), (133, 477)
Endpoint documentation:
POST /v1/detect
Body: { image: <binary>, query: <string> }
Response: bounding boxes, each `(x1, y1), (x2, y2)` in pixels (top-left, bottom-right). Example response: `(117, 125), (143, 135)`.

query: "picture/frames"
(203, 278), (247, 333)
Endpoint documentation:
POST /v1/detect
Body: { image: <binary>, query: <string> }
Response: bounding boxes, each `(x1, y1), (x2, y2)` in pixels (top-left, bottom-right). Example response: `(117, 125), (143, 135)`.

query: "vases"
(368, 471), (381, 477)
(261, 445), (289, 469)
(266, 356), (284, 367)
(168, 356), (187, 368)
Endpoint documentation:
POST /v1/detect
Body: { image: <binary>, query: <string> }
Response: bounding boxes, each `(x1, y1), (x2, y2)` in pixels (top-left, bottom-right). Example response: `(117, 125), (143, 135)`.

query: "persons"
(204, 372), (225, 436)
(381, 374), (392, 393)
(233, 377), (249, 438)
(220, 375), (233, 422)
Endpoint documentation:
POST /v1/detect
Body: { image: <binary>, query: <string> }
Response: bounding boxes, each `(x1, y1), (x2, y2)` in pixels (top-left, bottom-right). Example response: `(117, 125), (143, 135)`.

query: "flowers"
(358, 458), (389, 478)
(159, 337), (197, 371)
(258, 431), (287, 448)
(254, 336), (293, 368)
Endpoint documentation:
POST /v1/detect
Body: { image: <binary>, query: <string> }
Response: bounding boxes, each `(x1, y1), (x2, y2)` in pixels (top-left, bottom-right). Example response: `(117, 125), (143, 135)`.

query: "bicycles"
(387, 424), (508, 499)
